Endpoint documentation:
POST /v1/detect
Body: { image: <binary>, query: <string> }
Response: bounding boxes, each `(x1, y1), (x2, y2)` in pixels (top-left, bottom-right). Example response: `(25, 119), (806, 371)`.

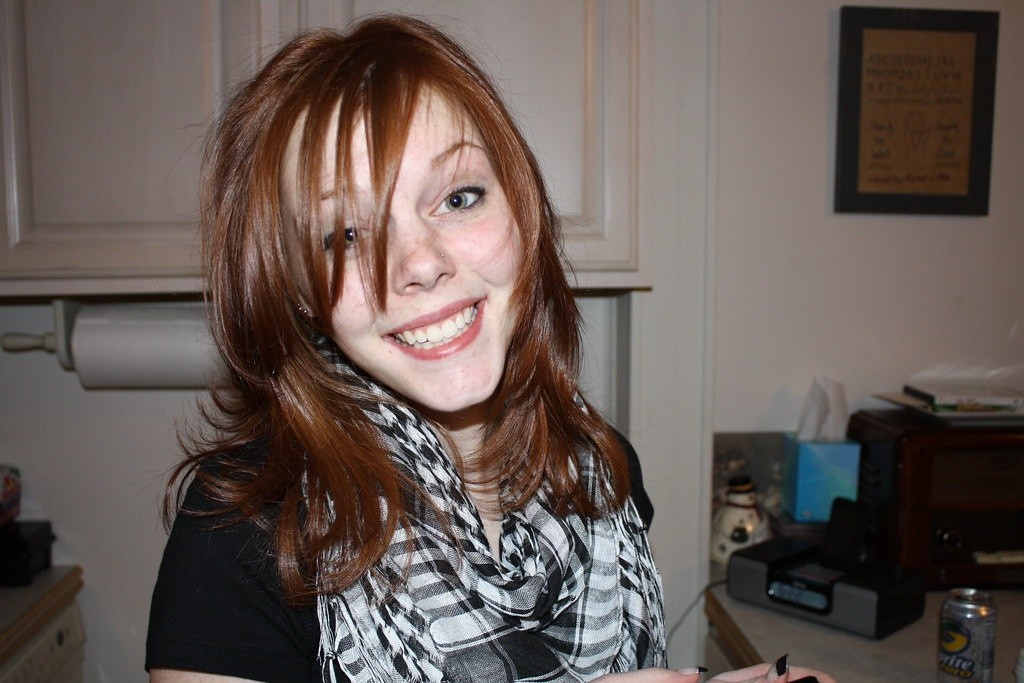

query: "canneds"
(936, 588), (997, 683)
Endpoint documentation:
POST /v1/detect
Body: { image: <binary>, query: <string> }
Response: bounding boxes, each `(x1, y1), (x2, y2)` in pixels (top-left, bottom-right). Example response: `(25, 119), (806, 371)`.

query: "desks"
(707, 561), (1024, 683)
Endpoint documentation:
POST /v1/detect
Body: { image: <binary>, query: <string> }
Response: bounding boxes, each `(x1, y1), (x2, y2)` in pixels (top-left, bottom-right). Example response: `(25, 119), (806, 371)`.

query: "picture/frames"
(833, 5), (999, 217)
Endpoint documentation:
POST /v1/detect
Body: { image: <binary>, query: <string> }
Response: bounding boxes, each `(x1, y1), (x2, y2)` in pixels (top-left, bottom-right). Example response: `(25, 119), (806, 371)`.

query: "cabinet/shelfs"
(0, 0), (655, 302)
(0, 564), (88, 683)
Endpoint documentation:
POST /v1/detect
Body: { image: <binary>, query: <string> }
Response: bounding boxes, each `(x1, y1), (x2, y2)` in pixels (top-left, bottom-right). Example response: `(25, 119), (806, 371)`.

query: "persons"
(144, 18), (839, 683)
